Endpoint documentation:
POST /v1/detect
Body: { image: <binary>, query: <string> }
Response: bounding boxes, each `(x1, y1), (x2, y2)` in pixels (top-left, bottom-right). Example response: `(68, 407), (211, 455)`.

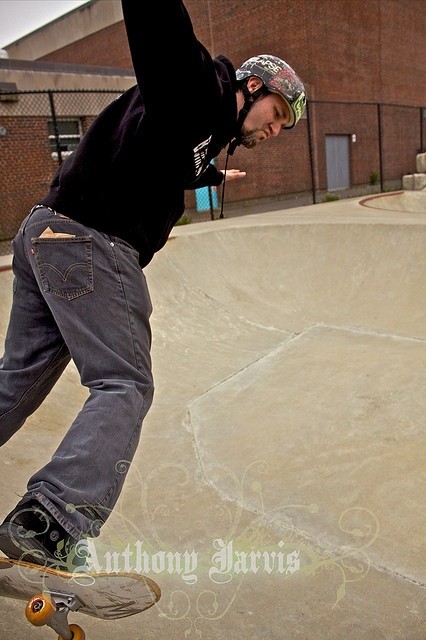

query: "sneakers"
(0, 493), (96, 573)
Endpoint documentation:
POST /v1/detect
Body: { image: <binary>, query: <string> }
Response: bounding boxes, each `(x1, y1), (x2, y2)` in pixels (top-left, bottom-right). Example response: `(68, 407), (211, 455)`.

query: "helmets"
(235, 54), (307, 129)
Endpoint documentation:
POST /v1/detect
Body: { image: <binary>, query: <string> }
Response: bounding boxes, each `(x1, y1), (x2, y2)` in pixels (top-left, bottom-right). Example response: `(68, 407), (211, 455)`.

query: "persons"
(0, 0), (306, 574)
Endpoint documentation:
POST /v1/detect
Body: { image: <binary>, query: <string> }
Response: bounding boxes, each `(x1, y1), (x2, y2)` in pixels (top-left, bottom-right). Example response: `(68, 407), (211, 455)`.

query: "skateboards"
(0, 556), (162, 640)
